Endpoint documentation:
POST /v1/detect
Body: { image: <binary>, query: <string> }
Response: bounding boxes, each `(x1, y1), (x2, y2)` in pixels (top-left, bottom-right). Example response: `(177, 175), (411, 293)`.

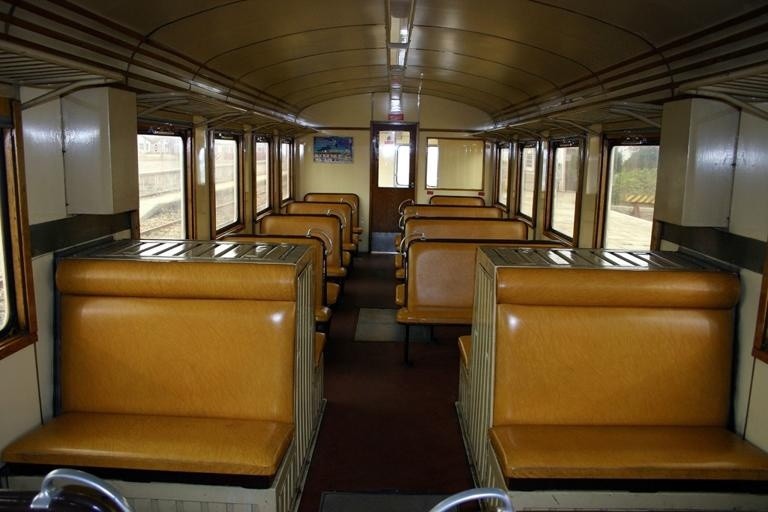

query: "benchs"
(0, 258), (301, 492)
(487, 267), (766, 495)
(216, 191), (365, 368)
(394, 195), (570, 371)
